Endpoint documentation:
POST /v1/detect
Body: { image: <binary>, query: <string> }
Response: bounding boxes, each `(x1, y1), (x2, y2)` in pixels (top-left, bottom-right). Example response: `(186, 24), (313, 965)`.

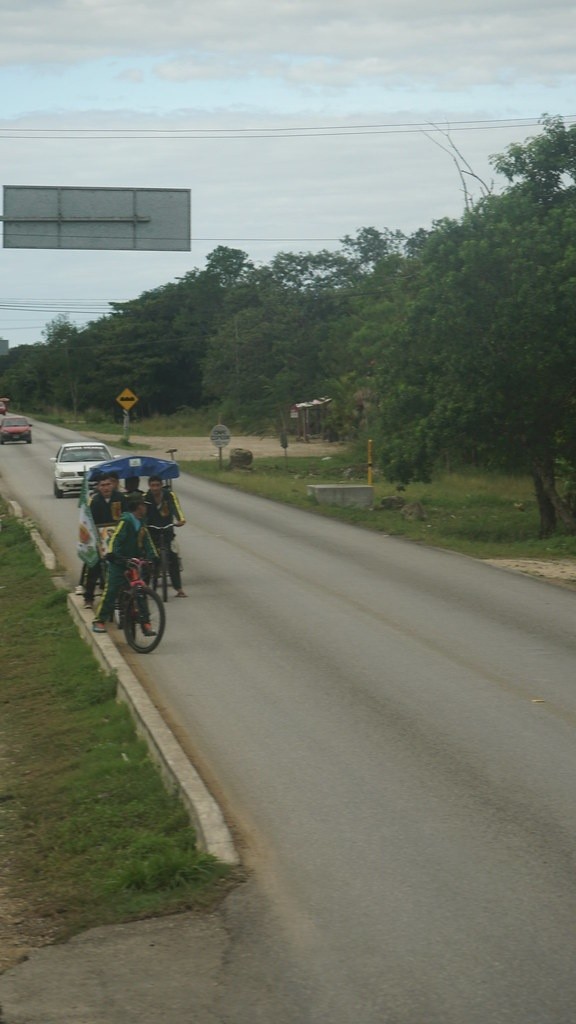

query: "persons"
(75, 472), (189, 609)
(92, 491), (159, 636)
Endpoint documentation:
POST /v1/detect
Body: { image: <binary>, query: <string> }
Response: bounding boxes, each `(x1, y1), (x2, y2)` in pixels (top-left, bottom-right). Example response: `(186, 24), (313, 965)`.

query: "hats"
(127, 492), (151, 505)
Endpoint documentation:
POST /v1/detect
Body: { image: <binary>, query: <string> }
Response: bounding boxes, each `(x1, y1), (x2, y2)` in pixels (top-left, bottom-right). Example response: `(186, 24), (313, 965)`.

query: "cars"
(49, 442), (123, 499)
(0, 417), (33, 444)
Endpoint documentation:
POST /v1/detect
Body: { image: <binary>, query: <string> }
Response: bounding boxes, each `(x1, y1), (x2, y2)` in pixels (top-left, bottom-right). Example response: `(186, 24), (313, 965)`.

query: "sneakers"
(92, 618), (107, 633)
(142, 622), (157, 636)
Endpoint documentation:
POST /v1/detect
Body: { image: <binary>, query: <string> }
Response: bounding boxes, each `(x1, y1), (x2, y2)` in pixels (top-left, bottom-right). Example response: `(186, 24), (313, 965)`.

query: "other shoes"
(85, 599), (93, 608)
(75, 585), (86, 595)
(175, 589), (189, 598)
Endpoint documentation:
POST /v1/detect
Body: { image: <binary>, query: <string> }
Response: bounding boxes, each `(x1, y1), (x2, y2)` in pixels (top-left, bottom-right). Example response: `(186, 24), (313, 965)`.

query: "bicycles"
(76, 544), (184, 657)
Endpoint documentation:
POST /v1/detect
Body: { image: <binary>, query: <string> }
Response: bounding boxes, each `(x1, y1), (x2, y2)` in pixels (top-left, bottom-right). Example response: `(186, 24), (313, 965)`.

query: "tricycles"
(82, 457), (191, 604)
